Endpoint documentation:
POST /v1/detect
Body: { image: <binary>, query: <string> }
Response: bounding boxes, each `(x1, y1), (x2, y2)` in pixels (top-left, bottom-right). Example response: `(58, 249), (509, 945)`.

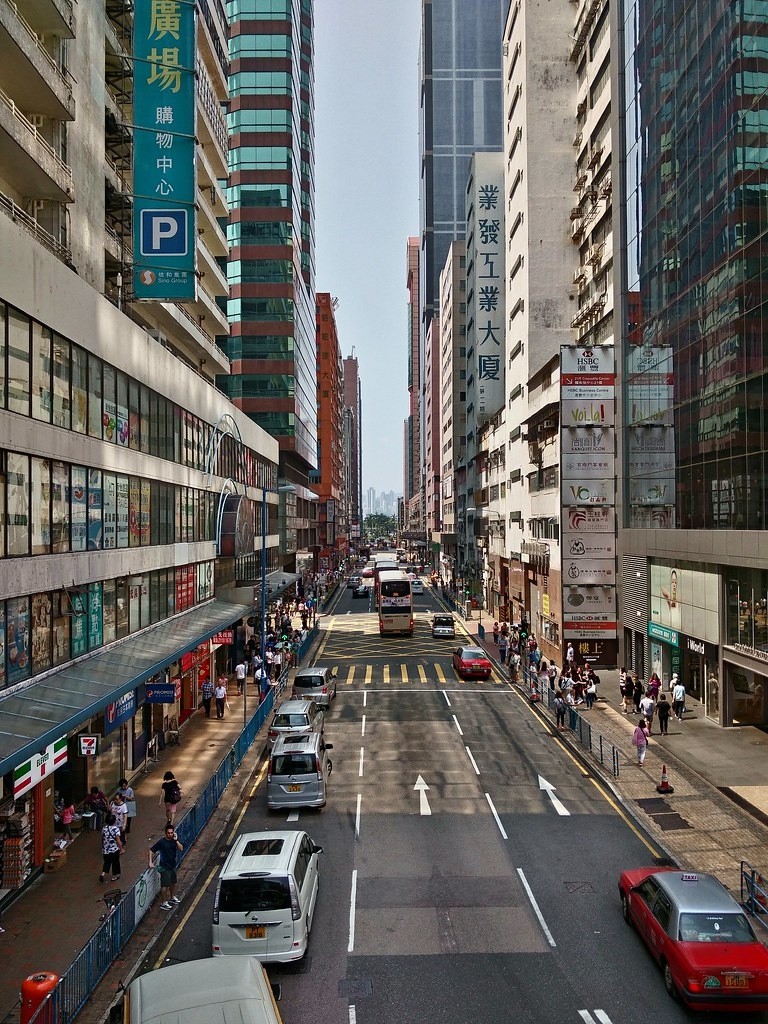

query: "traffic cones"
(529, 683), (539, 701)
(754, 874), (768, 913)
(656, 765), (675, 793)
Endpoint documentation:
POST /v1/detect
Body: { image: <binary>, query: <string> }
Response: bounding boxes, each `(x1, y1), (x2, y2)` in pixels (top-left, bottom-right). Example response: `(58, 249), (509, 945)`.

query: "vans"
(267, 700), (330, 748)
(431, 614), (456, 637)
(290, 667), (338, 709)
(266, 731), (333, 809)
(211, 830), (323, 963)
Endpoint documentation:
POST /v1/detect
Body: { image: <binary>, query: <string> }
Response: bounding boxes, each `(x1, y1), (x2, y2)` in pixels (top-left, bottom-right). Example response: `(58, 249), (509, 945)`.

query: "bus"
(374, 557), (423, 634)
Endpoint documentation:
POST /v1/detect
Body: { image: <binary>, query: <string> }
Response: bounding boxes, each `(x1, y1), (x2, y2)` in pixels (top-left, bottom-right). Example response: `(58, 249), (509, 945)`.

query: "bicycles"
(95, 889), (129, 968)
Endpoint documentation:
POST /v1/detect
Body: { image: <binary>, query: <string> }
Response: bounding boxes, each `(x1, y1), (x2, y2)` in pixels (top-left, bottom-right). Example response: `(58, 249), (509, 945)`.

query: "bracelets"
(174, 840), (179, 841)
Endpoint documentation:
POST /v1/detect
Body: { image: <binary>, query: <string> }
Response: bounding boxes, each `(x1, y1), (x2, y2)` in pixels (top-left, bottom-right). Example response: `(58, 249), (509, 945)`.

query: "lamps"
(571, 131), (612, 328)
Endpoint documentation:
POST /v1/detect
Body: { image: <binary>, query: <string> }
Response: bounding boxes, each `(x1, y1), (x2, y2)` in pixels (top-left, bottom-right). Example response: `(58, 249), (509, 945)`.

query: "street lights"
(259, 486), (296, 691)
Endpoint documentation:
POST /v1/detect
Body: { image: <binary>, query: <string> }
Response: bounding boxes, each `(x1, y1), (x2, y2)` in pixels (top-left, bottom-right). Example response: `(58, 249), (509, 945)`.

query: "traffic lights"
(466, 585), (470, 595)
(313, 590), (317, 603)
(281, 622), (288, 641)
(518, 620), (528, 639)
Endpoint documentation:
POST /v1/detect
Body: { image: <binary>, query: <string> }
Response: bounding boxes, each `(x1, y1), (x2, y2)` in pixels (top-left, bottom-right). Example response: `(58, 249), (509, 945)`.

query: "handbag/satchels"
(632, 733), (638, 746)
(125, 797), (137, 817)
(169, 787), (181, 804)
(682, 706), (686, 713)
(593, 676), (600, 683)
(582, 689), (585, 697)
(646, 741), (648, 745)
(647, 684), (654, 692)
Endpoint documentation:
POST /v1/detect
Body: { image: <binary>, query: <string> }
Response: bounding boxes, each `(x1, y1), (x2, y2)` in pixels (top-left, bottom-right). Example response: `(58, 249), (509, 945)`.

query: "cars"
(618, 866), (768, 1009)
(452, 646), (491, 676)
(355, 547), (376, 578)
(110, 957), (284, 1024)
(347, 577), (369, 598)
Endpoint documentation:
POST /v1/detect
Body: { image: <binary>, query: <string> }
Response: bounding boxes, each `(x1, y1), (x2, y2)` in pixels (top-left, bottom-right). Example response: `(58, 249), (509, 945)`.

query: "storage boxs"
(44, 849), (67, 873)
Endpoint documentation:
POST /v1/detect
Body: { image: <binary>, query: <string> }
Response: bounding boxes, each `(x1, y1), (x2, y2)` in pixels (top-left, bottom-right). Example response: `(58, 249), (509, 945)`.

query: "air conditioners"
(537, 419), (555, 433)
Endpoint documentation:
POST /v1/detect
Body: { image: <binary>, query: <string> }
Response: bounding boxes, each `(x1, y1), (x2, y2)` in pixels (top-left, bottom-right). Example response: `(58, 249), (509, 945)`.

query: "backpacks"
(561, 679), (569, 691)
(558, 677), (562, 688)
(557, 697), (565, 714)
(640, 685), (645, 694)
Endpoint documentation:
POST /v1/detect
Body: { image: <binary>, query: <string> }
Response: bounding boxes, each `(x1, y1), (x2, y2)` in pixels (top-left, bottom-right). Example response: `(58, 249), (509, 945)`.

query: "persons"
(661, 570), (682, 631)
(114, 778), (136, 842)
(707, 673), (720, 717)
(631, 718), (651, 766)
(107, 792), (127, 848)
(0, 926), (6, 933)
(199, 540), (687, 738)
(54, 799), (75, 843)
(148, 825), (184, 911)
(158, 771), (182, 828)
(99, 814), (126, 884)
(74, 786), (112, 831)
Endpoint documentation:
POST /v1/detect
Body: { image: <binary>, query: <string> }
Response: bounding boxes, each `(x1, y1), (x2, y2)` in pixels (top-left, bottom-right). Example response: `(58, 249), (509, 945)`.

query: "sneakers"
(160, 900), (172, 911)
(170, 896), (181, 904)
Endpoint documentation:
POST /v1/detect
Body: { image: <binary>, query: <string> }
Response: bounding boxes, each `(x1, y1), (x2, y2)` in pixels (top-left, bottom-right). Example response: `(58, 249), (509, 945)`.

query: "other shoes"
(622, 711), (628, 714)
(584, 707), (589, 710)
(575, 698), (583, 704)
(675, 713), (682, 721)
(619, 703), (624, 706)
(111, 875), (120, 881)
(639, 761), (643, 766)
(590, 707), (593, 709)
(99, 873), (104, 882)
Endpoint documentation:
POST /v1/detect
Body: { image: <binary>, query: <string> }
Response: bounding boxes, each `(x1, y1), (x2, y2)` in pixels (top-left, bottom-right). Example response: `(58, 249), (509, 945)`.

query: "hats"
(710, 673), (714, 678)
